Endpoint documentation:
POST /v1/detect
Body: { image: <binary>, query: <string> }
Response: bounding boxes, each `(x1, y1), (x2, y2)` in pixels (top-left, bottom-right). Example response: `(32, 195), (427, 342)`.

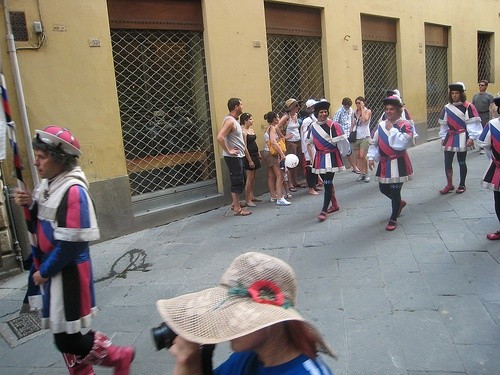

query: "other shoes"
(356, 174), (365, 182)
(318, 212), (326, 219)
(487, 231), (500, 240)
(365, 173), (370, 182)
(246, 182), (322, 206)
(386, 220), (397, 230)
(480, 149), (485, 153)
(456, 187), (466, 193)
(397, 200), (406, 216)
(440, 184), (454, 193)
(353, 168), (362, 174)
(327, 206), (340, 213)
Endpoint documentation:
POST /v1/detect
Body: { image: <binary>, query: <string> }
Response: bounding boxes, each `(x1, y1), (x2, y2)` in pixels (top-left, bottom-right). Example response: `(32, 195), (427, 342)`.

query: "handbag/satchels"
(348, 131), (357, 142)
(267, 129), (287, 155)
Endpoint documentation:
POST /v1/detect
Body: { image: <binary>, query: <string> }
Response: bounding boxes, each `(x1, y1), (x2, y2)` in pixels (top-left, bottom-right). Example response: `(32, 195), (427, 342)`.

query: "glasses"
(479, 85), (486, 87)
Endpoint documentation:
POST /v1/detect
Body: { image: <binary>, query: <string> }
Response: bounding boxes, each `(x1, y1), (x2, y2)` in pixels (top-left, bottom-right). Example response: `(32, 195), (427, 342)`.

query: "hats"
(448, 83), (464, 93)
(35, 126), (82, 156)
(285, 99), (303, 112)
(155, 252), (338, 359)
(306, 99), (318, 108)
(314, 101), (331, 111)
(383, 96), (401, 107)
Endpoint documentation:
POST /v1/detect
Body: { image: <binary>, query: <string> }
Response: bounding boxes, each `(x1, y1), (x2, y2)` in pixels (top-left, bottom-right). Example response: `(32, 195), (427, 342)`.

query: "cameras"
(151, 322), (177, 351)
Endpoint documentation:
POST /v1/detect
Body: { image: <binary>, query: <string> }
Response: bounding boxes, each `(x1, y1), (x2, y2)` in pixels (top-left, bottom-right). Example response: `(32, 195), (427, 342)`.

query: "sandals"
(231, 202), (245, 210)
(233, 208), (251, 215)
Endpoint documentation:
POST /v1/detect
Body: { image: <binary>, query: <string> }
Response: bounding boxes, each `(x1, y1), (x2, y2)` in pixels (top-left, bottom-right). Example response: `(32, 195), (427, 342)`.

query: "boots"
(73, 335), (135, 375)
(61, 350), (95, 375)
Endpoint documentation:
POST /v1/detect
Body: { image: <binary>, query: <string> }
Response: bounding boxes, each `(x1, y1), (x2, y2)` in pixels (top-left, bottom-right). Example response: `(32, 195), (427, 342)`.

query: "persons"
(16, 126), (135, 375)
(158, 252), (339, 375)
(216, 80), (500, 241)
